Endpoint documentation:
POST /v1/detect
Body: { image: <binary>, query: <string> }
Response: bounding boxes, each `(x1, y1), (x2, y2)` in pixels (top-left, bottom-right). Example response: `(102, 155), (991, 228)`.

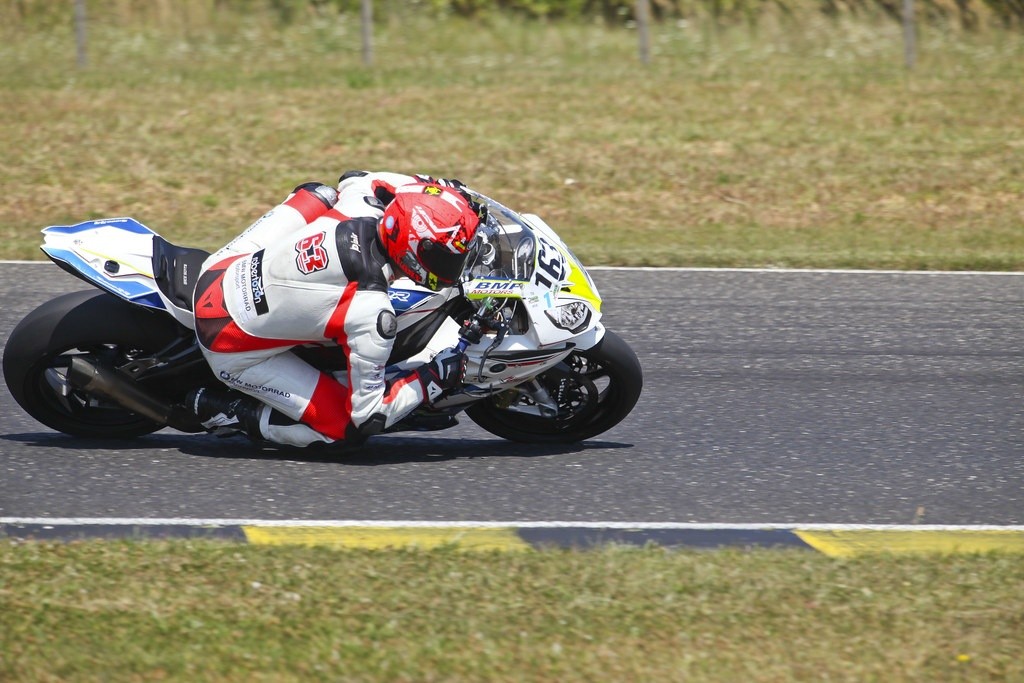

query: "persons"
(191, 165), (482, 454)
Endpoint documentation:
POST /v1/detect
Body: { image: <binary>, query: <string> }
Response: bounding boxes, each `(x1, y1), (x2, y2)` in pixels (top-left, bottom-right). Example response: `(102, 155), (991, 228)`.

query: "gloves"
(415, 347), (468, 402)
(416, 172), (469, 202)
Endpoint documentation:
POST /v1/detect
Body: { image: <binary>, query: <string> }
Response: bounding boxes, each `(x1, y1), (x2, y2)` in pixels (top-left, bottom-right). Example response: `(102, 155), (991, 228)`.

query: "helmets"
(377, 183), (479, 291)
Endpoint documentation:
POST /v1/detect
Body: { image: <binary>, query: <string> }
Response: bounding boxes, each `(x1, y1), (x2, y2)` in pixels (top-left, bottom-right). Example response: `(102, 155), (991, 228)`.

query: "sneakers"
(192, 386), (240, 438)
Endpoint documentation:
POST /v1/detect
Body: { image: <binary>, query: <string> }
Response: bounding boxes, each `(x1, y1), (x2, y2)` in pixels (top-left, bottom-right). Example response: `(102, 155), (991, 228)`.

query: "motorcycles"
(3, 187), (646, 451)
